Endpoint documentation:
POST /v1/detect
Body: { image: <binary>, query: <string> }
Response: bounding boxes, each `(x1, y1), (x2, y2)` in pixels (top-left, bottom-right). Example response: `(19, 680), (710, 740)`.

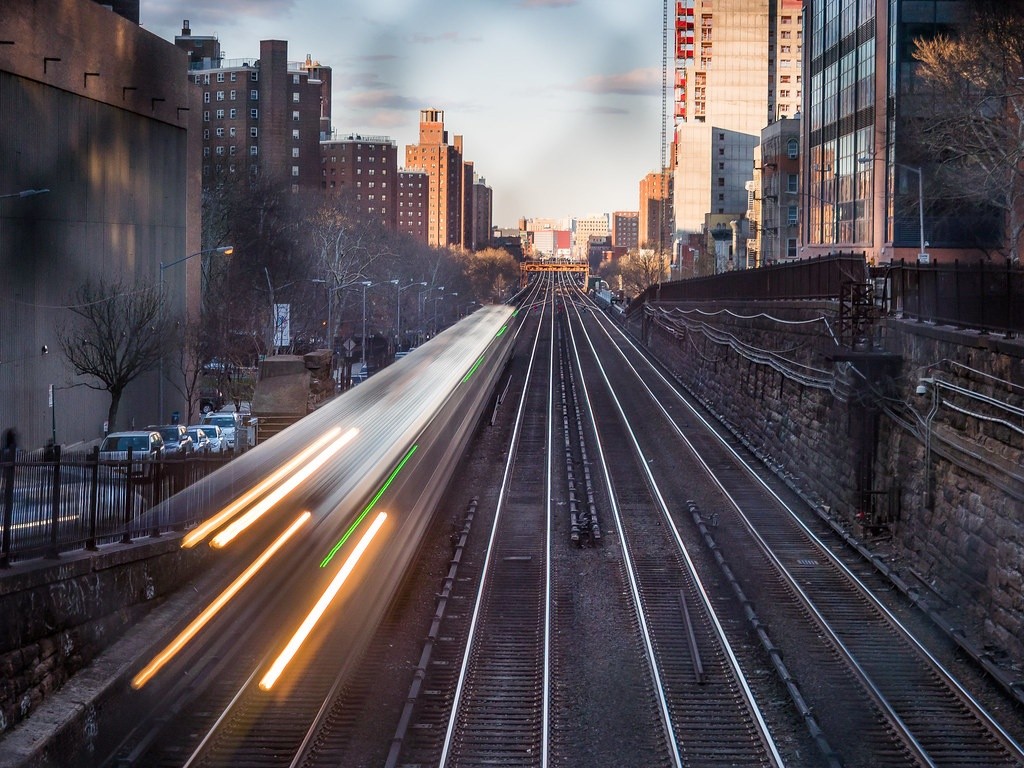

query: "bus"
(201, 412), (238, 451)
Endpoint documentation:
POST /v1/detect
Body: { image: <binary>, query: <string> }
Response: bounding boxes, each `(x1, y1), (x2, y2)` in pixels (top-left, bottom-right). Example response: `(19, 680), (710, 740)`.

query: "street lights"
(858, 158), (924, 254)
(269, 279), (325, 354)
(398, 282), (428, 351)
(363, 280), (399, 361)
(434, 292), (458, 336)
(158, 246), (233, 423)
(418, 286), (444, 346)
(328, 281), (371, 349)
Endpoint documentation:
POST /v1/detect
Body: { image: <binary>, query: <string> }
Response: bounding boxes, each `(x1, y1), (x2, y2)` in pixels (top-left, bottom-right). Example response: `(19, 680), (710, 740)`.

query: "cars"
(188, 429), (211, 456)
(99, 431), (165, 483)
(187, 425), (229, 456)
(142, 425), (194, 456)
